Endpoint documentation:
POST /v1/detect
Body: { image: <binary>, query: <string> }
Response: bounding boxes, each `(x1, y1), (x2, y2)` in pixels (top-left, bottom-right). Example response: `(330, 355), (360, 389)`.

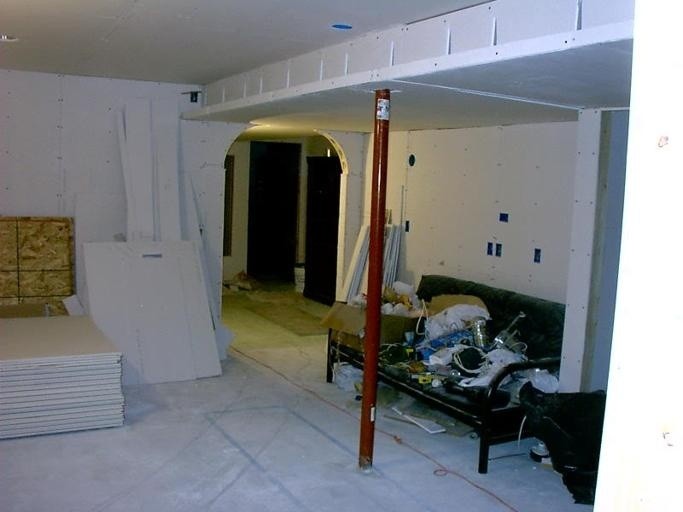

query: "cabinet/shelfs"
(303, 155), (341, 307)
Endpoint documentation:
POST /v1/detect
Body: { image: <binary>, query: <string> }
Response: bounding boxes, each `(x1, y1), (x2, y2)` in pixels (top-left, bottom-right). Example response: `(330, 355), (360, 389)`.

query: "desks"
(517, 381), (607, 512)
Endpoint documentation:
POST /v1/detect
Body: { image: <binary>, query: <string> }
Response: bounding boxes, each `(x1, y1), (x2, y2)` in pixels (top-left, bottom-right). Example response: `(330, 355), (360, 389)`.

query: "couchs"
(326, 274), (566, 474)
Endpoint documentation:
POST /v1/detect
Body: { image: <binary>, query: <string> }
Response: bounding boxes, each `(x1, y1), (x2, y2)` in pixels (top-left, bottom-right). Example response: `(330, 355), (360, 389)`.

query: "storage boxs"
(319, 301), (426, 353)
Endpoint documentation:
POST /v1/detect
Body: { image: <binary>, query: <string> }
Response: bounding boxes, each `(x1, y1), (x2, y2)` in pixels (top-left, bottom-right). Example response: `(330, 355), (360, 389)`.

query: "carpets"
(243, 296), (331, 336)
